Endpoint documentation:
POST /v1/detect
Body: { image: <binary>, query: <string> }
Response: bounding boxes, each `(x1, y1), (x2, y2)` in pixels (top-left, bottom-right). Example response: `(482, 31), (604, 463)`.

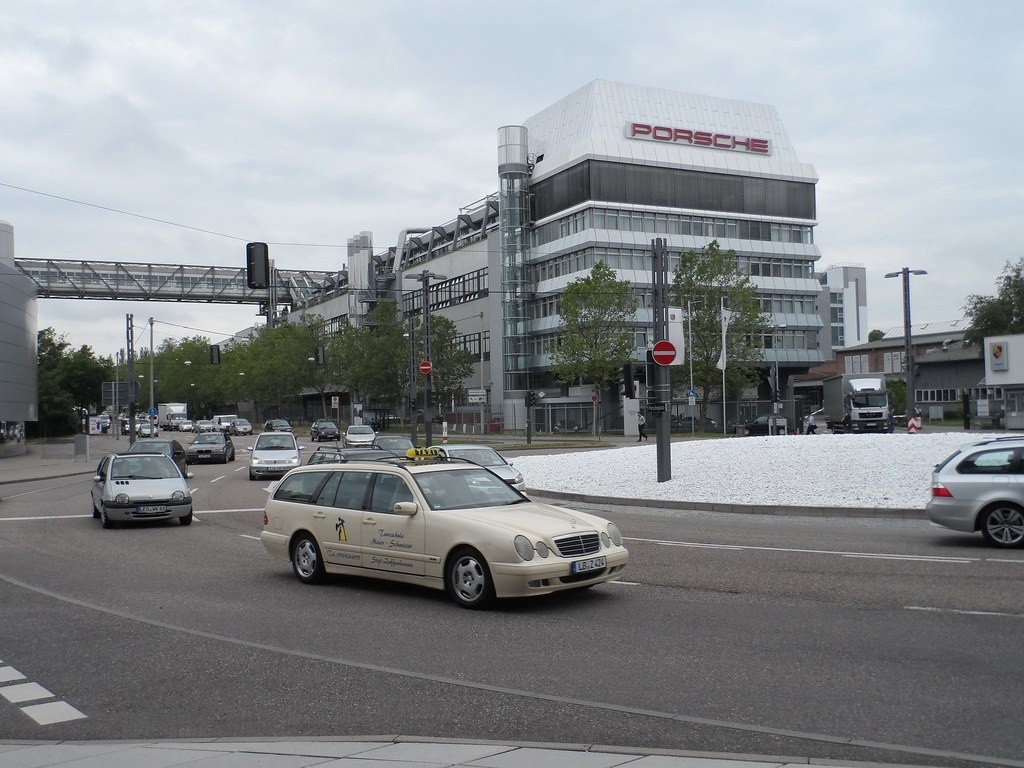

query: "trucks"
(158, 403), (187, 431)
(823, 372), (889, 434)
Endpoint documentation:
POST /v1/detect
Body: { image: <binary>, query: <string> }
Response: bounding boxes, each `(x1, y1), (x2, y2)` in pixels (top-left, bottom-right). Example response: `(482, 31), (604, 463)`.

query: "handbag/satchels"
(814, 424), (817, 429)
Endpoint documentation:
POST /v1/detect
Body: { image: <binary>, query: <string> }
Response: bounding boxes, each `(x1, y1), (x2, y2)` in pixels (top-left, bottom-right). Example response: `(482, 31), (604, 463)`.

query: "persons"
(276, 417), (279, 420)
(96, 420), (100, 428)
(842, 412), (853, 434)
(887, 409), (894, 433)
(916, 405), (921, 417)
(991, 344), (1004, 364)
(806, 412), (818, 435)
(370, 418), (381, 426)
(285, 416), (290, 424)
(637, 412), (648, 442)
(126, 413), (145, 419)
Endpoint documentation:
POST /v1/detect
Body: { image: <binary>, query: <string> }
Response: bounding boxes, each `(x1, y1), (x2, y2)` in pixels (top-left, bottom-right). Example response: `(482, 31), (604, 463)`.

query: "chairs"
(117, 460), (130, 474)
(200, 437), (207, 441)
(381, 477), (411, 513)
(279, 438), (288, 445)
(216, 436), (221, 440)
(427, 474), (463, 506)
(263, 441), (274, 446)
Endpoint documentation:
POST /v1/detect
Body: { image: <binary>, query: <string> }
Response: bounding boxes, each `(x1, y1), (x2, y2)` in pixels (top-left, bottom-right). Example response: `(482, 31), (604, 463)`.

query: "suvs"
(925, 435), (1024, 550)
(260, 443), (630, 606)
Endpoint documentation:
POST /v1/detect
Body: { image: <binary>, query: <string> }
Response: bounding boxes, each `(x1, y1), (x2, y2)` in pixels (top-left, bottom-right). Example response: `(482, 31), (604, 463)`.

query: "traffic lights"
(527, 361), (636, 407)
(774, 390), (781, 403)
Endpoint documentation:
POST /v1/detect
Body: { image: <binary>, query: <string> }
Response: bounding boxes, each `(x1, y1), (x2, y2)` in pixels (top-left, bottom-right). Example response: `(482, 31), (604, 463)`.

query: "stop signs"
(652, 341), (676, 366)
(418, 361), (432, 374)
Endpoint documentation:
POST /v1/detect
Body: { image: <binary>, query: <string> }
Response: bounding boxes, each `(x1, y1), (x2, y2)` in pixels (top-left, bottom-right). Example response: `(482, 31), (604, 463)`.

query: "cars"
(428, 444), (527, 499)
(121, 419), (158, 438)
(372, 435), (421, 460)
(735, 414), (790, 435)
(90, 432), (235, 527)
(179, 415), (237, 434)
(263, 420), (379, 449)
(246, 432), (305, 480)
(97, 411), (112, 428)
(381, 409), (504, 432)
(228, 419), (252, 436)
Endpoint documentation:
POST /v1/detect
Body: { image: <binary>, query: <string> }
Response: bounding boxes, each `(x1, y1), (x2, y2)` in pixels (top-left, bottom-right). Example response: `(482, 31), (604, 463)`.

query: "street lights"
(405, 270), (448, 447)
(688, 267), (928, 435)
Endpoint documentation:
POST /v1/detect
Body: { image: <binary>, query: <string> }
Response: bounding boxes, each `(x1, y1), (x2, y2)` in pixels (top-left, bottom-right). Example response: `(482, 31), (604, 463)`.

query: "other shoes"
(637, 440), (641, 442)
(646, 436), (648, 440)
